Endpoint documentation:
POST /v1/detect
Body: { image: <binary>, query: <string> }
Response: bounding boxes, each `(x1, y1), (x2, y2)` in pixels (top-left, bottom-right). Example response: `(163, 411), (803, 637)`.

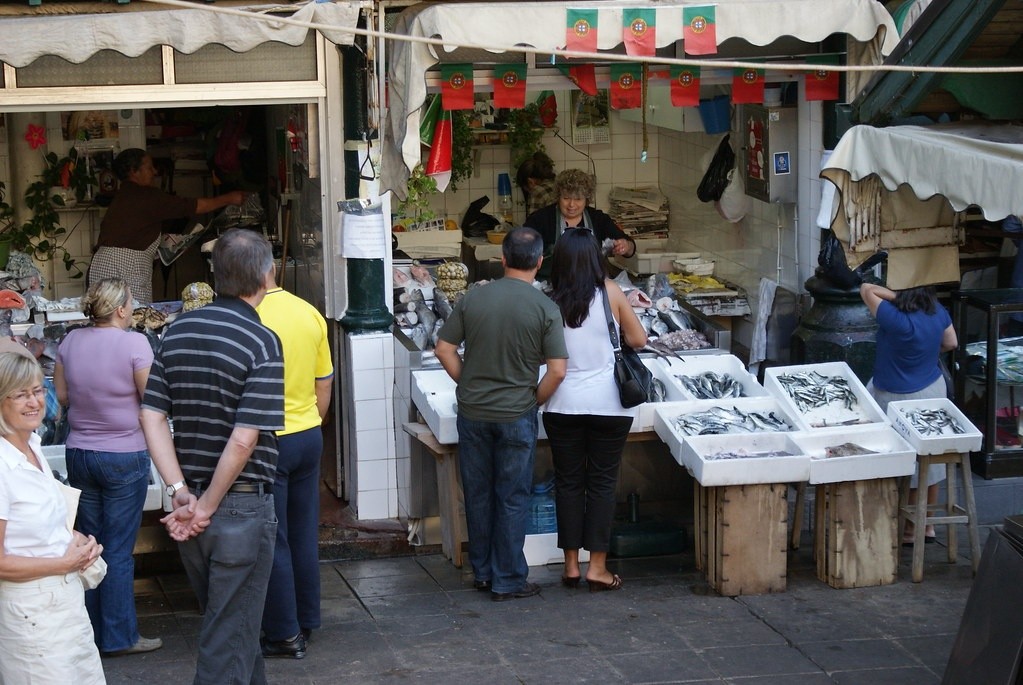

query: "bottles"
(528, 482), (557, 534)
(627, 491), (639, 522)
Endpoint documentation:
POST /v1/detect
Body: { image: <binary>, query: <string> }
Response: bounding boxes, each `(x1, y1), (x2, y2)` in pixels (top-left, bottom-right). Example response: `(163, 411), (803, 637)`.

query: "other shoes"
(104, 634), (162, 655)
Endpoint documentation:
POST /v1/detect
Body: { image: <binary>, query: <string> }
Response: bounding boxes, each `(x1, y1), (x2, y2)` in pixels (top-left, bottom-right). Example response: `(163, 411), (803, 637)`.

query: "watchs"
(166, 480), (187, 498)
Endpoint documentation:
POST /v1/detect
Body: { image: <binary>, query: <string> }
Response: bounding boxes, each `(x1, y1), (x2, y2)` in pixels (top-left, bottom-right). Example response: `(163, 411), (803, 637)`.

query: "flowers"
(24, 122), (52, 168)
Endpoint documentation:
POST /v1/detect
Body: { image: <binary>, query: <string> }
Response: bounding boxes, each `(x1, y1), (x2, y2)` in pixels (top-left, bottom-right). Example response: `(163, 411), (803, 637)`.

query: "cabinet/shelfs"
(949, 288), (1023, 481)
(471, 127), (561, 150)
(620, 86), (717, 132)
(959, 215), (1000, 258)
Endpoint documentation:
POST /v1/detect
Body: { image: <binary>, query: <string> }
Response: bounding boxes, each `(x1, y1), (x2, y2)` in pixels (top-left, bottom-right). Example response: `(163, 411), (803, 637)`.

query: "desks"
(462, 236), (503, 282)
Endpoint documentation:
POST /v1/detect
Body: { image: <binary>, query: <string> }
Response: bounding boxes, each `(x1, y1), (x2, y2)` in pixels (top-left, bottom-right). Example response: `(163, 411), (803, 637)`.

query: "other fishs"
(393, 261), (432, 287)
(673, 371), (748, 399)
(35, 409), (71, 487)
(671, 405), (791, 436)
(704, 448), (793, 460)
(623, 273), (694, 366)
(901, 406), (965, 436)
(649, 377), (667, 402)
(408, 288), (452, 349)
(826, 444), (879, 460)
(777, 371), (860, 426)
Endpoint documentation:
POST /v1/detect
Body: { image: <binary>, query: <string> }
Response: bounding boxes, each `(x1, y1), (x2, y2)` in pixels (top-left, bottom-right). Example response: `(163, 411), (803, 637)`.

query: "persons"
(859, 283), (958, 547)
(514, 151), (557, 218)
(533, 227), (646, 592)
(84, 147), (242, 304)
(524, 170), (636, 281)
(434, 227), (569, 603)
(0, 226), (334, 684)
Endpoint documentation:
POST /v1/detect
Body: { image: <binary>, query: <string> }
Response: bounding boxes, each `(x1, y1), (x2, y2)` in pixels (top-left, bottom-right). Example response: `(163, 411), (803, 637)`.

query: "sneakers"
(259, 629), (306, 658)
(300, 627), (311, 644)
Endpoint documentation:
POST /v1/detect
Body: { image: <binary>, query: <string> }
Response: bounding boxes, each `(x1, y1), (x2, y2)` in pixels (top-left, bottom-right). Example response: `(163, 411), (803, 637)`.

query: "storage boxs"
(392, 223), (463, 247)
(694, 477), (787, 597)
(615, 239), (700, 273)
(41, 444), (174, 512)
(815, 477), (898, 589)
(523, 532), (590, 566)
(411, 354), (983, 487)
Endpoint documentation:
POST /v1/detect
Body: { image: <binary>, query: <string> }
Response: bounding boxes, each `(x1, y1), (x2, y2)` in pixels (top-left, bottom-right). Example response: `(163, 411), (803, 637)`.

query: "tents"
(379, 0), (902, 205)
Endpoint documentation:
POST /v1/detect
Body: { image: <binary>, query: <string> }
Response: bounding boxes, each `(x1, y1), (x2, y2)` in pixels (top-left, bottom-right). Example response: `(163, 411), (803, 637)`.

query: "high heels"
(587, 572), (623, 593)
(560, 573), (581, 588)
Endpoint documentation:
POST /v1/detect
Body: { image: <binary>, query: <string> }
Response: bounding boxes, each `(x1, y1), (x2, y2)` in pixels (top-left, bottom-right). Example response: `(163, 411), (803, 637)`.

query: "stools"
(898, 451), (983, 584)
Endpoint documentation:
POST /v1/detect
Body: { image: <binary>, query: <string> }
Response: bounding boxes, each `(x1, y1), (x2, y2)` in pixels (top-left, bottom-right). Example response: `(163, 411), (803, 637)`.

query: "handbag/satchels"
(600, 280), (652, 408)
(716, 165), (749, 225)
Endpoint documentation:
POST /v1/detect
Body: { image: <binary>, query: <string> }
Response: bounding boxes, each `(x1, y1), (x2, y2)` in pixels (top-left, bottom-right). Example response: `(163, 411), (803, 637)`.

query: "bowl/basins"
(486, 230), (507, 243)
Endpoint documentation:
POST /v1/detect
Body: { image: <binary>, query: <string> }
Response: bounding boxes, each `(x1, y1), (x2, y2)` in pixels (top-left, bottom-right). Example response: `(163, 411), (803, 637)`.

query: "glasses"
(565, 226), (592, 235)
(6, 388), (47, 404)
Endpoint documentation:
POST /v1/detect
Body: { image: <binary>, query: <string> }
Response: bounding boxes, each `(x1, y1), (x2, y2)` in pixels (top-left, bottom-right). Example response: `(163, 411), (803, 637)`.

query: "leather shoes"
(490, 582), (540, 602)
(473, 579), (492, 589)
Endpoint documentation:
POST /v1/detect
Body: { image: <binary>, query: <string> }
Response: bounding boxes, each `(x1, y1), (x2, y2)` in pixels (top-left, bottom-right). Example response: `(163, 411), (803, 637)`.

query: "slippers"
(901, 540), (916, 548)
(925, 535), (935, 544)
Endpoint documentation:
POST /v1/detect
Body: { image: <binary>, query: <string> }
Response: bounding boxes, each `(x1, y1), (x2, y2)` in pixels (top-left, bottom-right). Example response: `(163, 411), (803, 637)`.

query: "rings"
(622, 248), (624, 252)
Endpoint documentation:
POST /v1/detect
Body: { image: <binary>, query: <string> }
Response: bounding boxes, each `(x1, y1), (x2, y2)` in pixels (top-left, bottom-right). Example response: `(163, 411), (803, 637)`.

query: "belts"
(184, 478), (268, 492)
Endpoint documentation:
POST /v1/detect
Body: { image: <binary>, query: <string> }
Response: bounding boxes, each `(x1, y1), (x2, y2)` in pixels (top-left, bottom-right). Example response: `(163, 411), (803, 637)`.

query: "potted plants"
(0, 147), (99, 278)
